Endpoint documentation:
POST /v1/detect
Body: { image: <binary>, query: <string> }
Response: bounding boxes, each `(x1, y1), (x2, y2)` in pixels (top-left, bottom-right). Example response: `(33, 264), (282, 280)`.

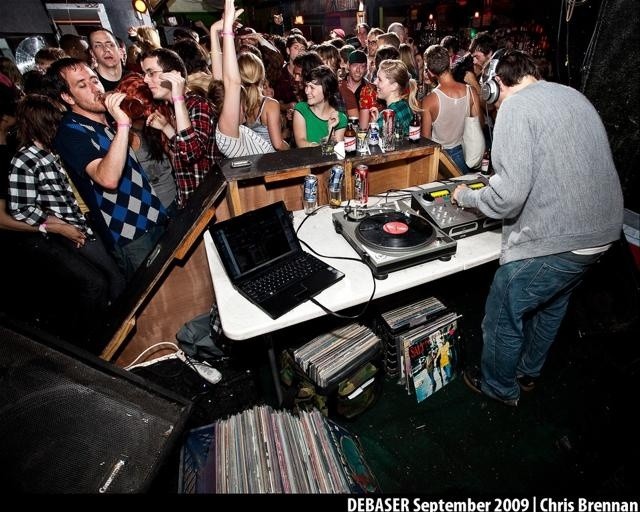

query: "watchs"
(220, 31), (235, 37)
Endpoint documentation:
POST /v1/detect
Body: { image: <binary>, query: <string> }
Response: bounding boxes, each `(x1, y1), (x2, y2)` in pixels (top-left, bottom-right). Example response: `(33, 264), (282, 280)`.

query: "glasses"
(141, 70), (162, 78)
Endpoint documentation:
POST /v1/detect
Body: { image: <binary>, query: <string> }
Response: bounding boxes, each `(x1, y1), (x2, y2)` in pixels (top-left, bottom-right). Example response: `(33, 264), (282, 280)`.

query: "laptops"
(207, 200), (346, 320)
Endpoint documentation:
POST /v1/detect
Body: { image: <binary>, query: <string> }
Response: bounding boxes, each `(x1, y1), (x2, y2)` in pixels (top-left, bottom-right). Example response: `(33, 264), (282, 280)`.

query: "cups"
(320, 137), (336, 154)
(328, 192), (341, 206)
(304, 201), (316, 215)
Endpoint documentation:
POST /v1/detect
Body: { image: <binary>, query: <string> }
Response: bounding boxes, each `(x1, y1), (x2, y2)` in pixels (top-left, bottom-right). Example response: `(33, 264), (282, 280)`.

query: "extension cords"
(177, 349), (223, 385)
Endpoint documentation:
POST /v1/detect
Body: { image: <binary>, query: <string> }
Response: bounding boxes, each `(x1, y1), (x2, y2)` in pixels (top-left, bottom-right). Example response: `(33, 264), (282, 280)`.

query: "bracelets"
(117, 122), (132, 128)
(172, 96), (185, 102)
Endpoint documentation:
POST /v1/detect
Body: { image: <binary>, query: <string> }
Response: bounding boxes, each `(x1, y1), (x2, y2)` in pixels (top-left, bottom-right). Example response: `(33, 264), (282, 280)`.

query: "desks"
(215, 133), (441, 216)
(204, 172), (509, 406)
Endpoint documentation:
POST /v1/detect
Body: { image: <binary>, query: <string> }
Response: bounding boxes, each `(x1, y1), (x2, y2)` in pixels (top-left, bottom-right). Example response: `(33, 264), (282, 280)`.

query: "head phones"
(479, 46), (530, 106)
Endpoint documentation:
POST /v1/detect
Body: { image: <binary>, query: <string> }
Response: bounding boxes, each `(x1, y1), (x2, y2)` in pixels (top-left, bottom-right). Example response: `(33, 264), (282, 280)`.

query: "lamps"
(132, 0), (148, 13)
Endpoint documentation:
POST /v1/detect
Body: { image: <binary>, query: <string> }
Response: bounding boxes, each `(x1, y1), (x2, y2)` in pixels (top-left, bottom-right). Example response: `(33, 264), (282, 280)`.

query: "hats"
(348, 50), (367, 64)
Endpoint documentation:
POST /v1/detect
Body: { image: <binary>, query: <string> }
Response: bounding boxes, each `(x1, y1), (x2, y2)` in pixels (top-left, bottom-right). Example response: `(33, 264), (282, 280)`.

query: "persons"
(111, 71), (178, 216)
(369, 59), (424, 138)
(188, 0), (276, 158)
(272, 13), (303, 50)
(421, 44), (481, 175)
(86, 26), (132, 92)
(293, 64), (349, 148)
(235, 27), (283, 82)
(48, 57), (169, 274)
(340, 49), (370, 112)
(127, 25), (213, 93)
(0, 31), (90, 95)
(139, 48), (223, 210)
(358, 45), (411, 130)
(463, 32), (500, 112)
(279, 33), (308, 113)
(237, 52), (290, 151)
(309, 13), (424, 68)
(450, 48), (624, 406)
(286, 51), (325, 121)
(0, 81), (86, 247)
(8, 98), (123, 279)
(440, 36), (461, 68)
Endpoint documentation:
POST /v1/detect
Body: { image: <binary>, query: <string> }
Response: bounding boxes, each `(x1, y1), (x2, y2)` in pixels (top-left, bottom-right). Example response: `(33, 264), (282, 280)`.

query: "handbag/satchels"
(462, 84), (486, 169)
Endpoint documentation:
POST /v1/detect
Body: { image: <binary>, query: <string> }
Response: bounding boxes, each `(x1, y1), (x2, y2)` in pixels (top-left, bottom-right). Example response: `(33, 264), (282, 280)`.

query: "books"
(213, 405), (384, 496)
(379, 295), (464, 406)
(292, 320), (384, 387)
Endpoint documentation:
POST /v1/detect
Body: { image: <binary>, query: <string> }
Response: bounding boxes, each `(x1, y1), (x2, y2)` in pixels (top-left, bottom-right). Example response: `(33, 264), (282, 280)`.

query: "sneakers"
(463, 364), (520, 406)
(518, 370), (535, 392)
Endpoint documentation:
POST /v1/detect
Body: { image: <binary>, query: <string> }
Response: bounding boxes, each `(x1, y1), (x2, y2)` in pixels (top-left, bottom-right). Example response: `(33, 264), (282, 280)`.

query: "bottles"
(382, 109), (395, 135)
(354, 164), (369, 204)
(368, 122), (379, 145)
(481, 149), (492, 175)
(344, 119), (356, 156)
(394, 121), (402, 146)
(329, 164), (344, 193)
(408, 111), (421, 143)
(357, 130), (368, 153)
(303, 173), (318, 202)
